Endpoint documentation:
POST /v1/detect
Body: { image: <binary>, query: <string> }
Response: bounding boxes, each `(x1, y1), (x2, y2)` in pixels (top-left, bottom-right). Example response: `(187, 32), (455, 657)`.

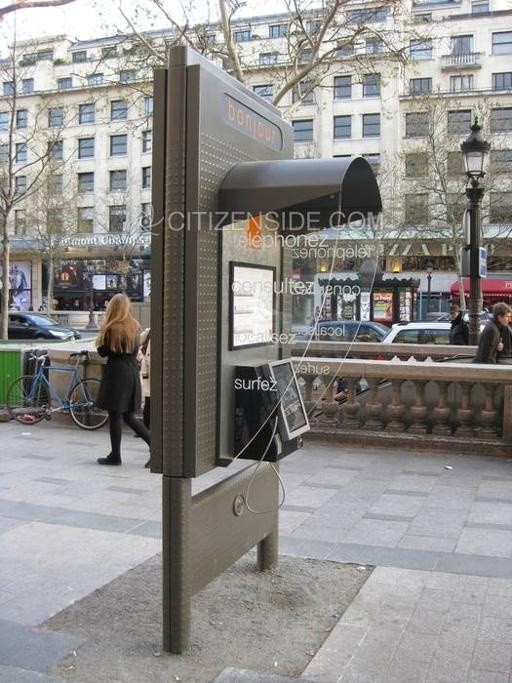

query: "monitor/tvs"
(261, 359), (310, 442)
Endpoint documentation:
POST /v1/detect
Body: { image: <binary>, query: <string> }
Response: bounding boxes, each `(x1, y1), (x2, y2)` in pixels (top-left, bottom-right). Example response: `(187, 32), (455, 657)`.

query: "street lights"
(459, 115), (492, 346)
(426, 261), (434, 311)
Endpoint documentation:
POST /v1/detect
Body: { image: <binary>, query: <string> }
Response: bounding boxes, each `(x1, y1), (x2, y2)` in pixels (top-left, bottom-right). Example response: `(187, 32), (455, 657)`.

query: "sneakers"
(98, 452), (122, 464)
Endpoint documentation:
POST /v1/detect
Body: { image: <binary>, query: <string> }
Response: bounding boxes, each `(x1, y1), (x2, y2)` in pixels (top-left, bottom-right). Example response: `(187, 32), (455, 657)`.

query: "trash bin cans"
(0, 343), (47, 409)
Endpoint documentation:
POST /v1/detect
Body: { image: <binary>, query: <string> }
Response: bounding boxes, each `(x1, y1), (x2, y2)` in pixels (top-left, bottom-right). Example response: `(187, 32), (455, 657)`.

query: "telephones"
(234, 359), (311, 463)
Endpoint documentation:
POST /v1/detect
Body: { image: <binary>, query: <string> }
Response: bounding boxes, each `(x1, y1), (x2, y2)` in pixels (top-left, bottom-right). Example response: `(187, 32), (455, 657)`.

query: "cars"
(7, 311), (81, 342)
(289, 309), (493, 360)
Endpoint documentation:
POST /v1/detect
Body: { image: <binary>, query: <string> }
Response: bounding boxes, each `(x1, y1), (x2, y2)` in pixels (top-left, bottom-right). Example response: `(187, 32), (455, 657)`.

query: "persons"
(134, 327), (151, 437)
(472, 302), (512, 364)
(448, 305), (469, 344)
(95, 294), (151, 468)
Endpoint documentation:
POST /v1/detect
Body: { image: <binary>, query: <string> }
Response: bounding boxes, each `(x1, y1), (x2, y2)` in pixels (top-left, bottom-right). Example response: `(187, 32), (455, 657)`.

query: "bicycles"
(6, 349), (110, 430)
